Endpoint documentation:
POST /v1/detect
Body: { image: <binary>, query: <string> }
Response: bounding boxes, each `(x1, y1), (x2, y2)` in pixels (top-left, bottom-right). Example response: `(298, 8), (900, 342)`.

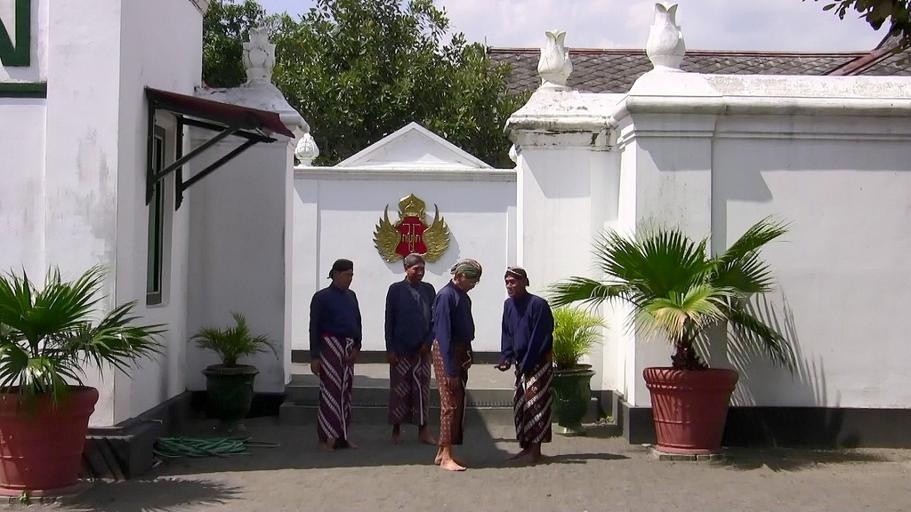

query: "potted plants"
(0, 257), (169, 493)
(187, 308), (283, 439)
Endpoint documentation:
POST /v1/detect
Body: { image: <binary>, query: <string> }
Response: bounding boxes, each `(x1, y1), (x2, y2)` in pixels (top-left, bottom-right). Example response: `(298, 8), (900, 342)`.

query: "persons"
(384, 253), (438, 445)
(307, 257), (362, 451)
(431, 259), (484, 474)
(498, 267), (554, 465)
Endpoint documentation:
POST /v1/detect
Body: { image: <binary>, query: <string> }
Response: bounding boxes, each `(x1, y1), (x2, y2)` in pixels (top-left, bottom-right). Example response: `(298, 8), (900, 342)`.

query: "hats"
(450, 258), (482, 278)
(328, 259), (353, 276)
(506, 266), (527, 279)
(404, 253), (425, 268)
